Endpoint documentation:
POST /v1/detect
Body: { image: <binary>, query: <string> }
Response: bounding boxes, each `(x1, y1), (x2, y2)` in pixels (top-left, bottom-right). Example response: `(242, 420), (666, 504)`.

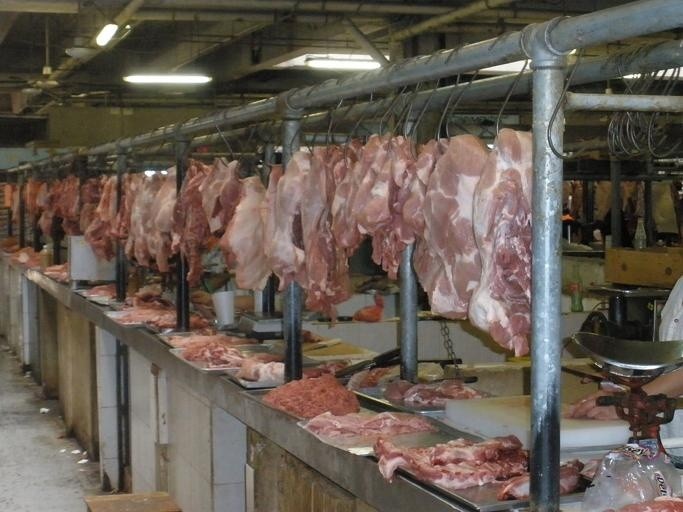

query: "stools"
(82, 491), (181, 512)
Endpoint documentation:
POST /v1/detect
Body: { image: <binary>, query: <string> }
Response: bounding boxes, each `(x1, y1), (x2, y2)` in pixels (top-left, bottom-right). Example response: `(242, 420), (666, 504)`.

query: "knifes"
(300, 338), (341, 352)
(329, 348), (401, 381)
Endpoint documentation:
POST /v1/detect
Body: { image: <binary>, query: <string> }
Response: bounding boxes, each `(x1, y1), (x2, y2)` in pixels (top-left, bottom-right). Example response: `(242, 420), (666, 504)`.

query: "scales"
(238, 311), (284, 339)
(572, 332), (683, 512)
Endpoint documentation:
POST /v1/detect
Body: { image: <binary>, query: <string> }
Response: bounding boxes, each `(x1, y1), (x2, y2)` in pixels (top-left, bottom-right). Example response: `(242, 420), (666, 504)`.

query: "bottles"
(634, 214), (649, 251)
(570, 285), (585, 312)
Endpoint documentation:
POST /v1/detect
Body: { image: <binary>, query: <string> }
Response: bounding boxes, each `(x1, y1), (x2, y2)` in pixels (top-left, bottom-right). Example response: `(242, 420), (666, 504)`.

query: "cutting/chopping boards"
(445, 394), (667, 451)
(270, 338), (378, 361)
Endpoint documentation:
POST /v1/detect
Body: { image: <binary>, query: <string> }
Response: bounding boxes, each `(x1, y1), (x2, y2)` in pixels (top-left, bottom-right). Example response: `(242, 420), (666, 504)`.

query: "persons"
(563, 271), (682, 441)
(190, 267), (254, 312)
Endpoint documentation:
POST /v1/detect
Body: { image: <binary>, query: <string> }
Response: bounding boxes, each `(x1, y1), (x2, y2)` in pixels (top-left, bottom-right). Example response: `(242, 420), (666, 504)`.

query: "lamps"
(121, 71), (215, 90)
(93, 22), (121, 48)
(302, 51), (379, 71)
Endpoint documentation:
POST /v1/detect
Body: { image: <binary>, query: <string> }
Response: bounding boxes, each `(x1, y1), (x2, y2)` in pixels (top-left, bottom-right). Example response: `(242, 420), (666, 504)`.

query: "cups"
(211, 291), (236, 325)
